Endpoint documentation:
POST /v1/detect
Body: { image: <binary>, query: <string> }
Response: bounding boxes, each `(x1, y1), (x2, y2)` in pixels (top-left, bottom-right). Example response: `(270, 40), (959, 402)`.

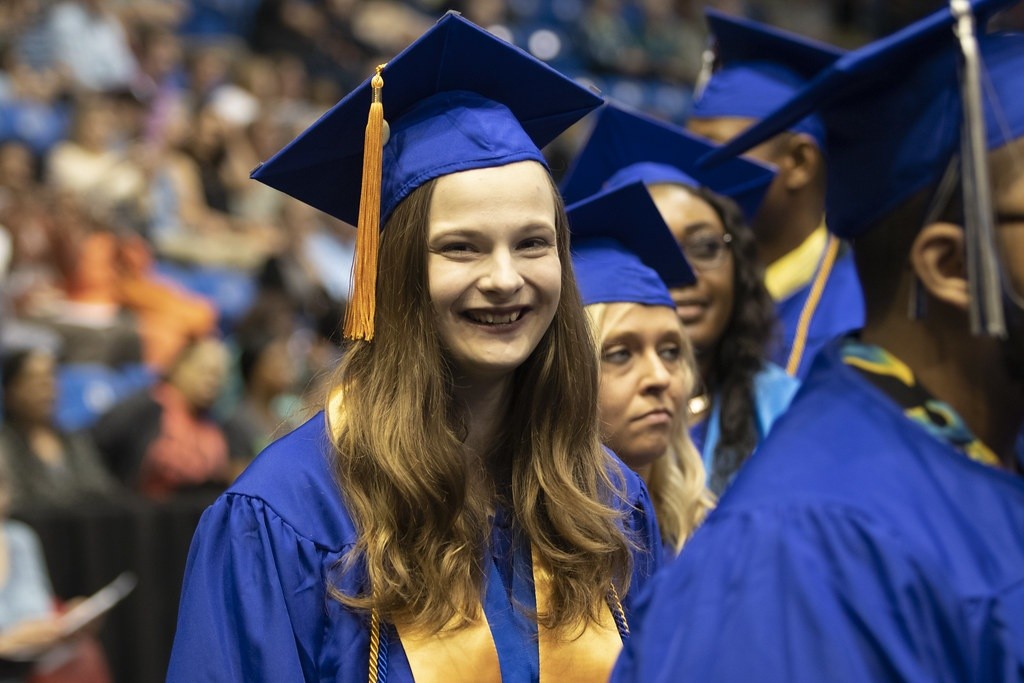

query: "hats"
(682, 9), (855, 149)
(560, 97), (777, 223)
(562, 179), (697, 305)
(721, 1), (1024, 338)
(249, 11), (604, 344)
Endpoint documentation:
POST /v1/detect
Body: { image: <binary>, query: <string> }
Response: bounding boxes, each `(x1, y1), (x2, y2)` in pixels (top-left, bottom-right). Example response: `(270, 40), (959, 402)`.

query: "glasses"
(685, 232), (733, 268)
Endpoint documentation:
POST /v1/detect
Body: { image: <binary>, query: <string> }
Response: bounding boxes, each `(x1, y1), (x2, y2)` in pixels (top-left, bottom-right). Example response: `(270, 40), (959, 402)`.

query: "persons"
(569, 101), (799, 499)
(565, 175), (719, 562)
(0, 0), (771, 683)
(608, 0), (1024, 683)
(162, 10), (668, 683)
(677, 7), (869, 381)
